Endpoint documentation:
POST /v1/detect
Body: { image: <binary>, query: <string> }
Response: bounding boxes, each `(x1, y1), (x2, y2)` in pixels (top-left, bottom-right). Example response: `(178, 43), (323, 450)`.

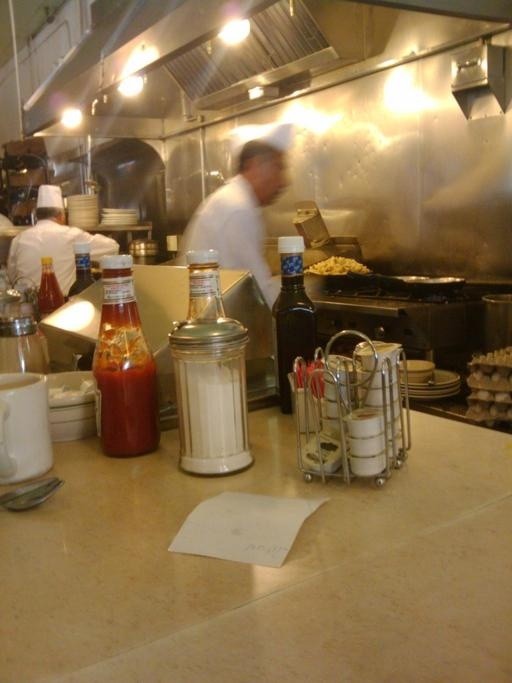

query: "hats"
(36, 184), (64, 209)
(235, 122), (293, 151)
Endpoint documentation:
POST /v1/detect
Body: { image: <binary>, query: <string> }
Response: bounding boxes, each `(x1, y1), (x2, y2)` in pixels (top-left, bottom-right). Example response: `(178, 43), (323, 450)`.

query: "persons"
(6, 206), (120, 298)
(177, 140), (292, 313)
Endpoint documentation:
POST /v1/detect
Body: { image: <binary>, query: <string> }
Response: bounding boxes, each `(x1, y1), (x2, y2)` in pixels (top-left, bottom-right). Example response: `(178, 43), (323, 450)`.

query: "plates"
(67, 194), (138, 227)
(400, 370), (462, 400)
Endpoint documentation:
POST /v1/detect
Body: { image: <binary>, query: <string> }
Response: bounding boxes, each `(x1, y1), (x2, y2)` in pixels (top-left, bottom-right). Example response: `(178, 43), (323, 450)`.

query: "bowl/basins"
(399, 359), (435, 382)
(47, 370), (96, 441)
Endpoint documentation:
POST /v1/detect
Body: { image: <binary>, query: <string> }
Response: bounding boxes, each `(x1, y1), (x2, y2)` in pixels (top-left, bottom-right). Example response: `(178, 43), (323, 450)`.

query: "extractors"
(20, 0), (511, 140)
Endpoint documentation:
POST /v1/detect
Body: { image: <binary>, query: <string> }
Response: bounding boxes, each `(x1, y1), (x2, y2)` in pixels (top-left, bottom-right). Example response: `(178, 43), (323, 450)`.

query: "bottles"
(272, 235), (315, 417)
(93, 255), (160, 458)
(167, 249), (256, 479)
(0, 240), (92, 372)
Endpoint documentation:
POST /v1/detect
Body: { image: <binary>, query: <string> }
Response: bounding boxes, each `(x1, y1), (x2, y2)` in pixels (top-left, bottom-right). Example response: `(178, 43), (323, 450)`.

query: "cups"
(0, 372), (55, 484)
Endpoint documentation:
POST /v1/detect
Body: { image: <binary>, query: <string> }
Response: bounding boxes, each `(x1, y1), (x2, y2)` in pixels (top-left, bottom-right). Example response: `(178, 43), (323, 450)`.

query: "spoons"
(0, 480), (65, 512)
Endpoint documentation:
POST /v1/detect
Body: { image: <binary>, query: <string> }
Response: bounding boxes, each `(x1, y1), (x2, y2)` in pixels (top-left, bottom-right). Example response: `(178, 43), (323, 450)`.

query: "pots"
(481, 294), (510, 351)
(306, 266), (466, 298)
